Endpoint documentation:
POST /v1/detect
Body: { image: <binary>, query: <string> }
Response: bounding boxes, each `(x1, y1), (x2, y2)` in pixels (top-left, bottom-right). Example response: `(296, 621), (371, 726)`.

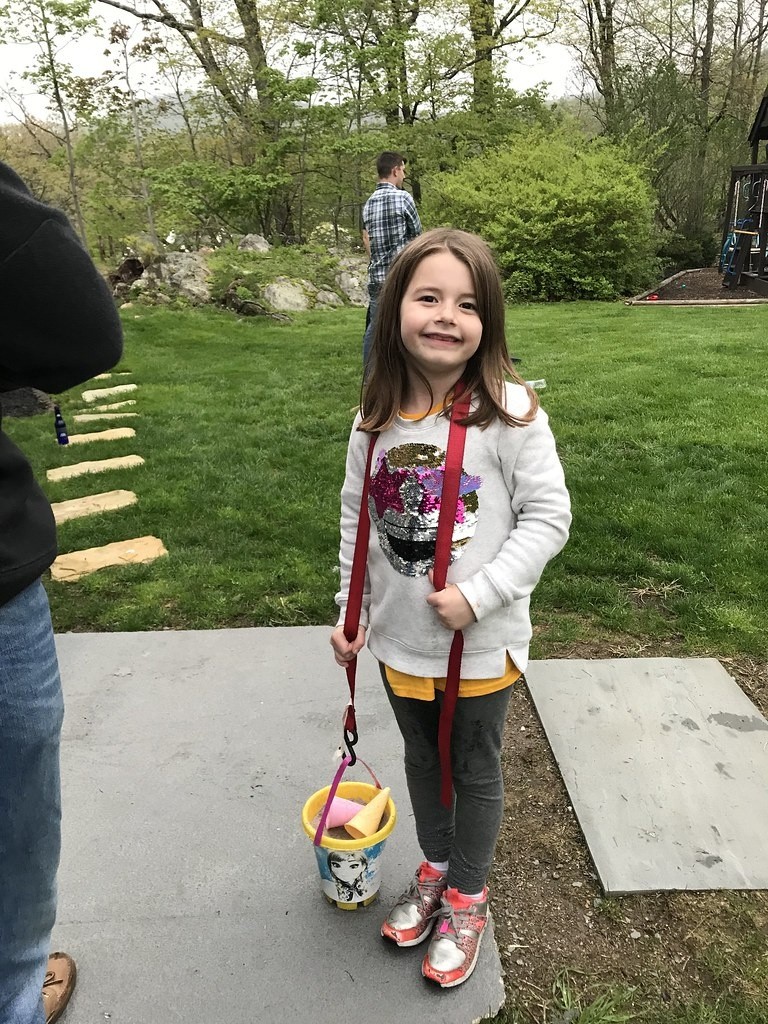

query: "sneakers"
(421, 885), (489, 989)
(39, 953), (77, 1024)
(380, 861), (449, 948)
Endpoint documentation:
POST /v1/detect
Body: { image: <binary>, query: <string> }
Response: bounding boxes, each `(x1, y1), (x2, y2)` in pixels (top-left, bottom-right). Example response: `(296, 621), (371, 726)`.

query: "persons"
(328, 228), (572, 991)
(0, 159), (124, 1024)
(358, 146), (424, 398)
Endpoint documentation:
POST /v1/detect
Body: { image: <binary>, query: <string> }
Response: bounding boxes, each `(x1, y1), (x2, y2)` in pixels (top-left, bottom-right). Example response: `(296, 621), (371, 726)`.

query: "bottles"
(54, 406), (68, 445)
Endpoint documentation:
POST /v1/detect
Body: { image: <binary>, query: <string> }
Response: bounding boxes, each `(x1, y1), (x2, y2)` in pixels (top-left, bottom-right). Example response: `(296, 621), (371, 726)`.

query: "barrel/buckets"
(301, 754), (396, 912)
